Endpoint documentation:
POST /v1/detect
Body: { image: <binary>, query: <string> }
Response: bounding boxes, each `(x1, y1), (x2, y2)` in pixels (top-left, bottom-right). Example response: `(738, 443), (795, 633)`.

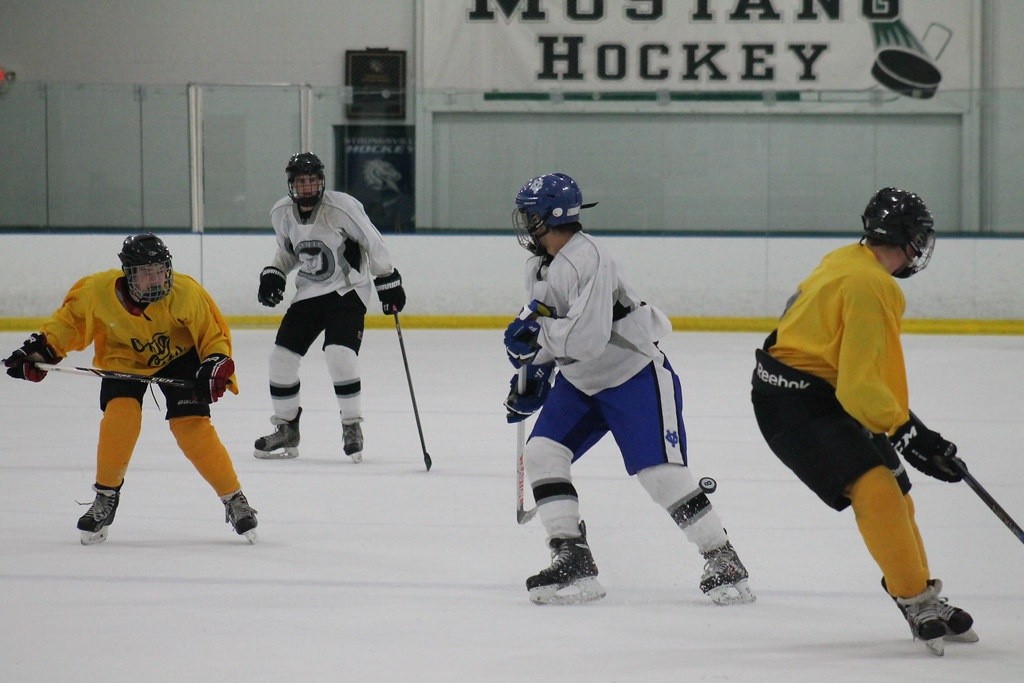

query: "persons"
(504, 172), (756, 606)
(252, 152), (405, 464)
(750, 187), (980, 658)
(5, 232), (259, 544)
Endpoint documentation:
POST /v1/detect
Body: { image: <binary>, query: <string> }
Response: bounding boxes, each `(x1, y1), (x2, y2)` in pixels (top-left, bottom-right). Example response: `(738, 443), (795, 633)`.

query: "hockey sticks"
(2, 359), (184, 387)
(909, 410), (1024, 542)
(516, 362), (528, 524)
(392, 304), (432, 472)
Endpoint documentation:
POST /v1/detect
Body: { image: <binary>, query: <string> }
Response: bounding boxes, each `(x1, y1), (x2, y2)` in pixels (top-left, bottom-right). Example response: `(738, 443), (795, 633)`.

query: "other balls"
(698, 477), (717, 494)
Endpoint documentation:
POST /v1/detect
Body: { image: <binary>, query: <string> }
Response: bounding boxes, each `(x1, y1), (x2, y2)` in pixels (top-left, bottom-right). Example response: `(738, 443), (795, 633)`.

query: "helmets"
(862, 184), (936, 279)
(119, 232), (173, 303)
(512, 172), (584, 256)
(285, 152), (326, 207)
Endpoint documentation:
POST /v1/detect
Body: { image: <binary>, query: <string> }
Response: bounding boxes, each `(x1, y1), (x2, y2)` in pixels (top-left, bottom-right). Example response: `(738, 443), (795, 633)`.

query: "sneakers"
(339, 409), (364, 462)
(929, 577), (979, 642)
(881, 576), (949, 656)
(525, 519), (606, 607)
(253, 406), (302, 458)
(699, 527), (755, 606)
(223, 490), (258, 543)
(73, 485), (120, 545)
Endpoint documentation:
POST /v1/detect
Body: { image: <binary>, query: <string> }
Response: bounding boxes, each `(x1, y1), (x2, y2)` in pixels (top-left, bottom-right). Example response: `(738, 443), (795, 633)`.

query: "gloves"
(504, 362), (551, 425)
(6, 331), (62, 382)
(257, 266), (286, 307)
(195, 353), (235, 404)
(374, 268), (406, 314)
(504, 299), (555, 368)
(886, 409), (968, 485)
